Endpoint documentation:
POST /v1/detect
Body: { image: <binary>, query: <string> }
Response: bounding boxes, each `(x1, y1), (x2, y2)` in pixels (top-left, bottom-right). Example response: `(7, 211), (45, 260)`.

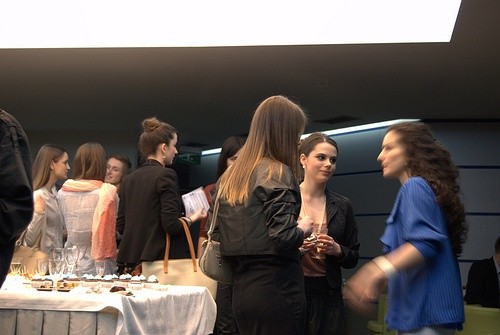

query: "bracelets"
(373, 255), (397, 279)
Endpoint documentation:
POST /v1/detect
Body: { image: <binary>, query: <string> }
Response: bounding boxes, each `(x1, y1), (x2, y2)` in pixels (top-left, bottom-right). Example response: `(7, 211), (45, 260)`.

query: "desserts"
(31, 272), (158, 292)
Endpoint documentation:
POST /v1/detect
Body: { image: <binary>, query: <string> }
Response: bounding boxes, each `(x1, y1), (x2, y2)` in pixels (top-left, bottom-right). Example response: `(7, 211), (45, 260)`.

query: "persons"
(0, 111), (34, 289)
(217, 95), (314, 335)
(57, 141), (119, 276)
(345, 119), (467, 335)
(466, 237), (500, 308)
(104, 116), (247, 283)
(296, 133), (360, 335)
(23, 145), (71, 275)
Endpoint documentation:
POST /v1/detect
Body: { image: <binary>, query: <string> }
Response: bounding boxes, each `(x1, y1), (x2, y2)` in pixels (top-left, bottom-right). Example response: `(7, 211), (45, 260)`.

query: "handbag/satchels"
(141, 216), (218, 301)
(9, 228), (49, 277)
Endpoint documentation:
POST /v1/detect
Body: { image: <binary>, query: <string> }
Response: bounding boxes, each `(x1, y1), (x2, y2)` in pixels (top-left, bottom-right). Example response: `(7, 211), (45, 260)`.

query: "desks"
(0, 287), (218, 335)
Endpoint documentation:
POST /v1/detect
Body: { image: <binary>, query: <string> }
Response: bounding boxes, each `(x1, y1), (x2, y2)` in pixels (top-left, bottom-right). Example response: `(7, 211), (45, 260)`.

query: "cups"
(53, 248), (64, 261)
(95, 261), (105, 277)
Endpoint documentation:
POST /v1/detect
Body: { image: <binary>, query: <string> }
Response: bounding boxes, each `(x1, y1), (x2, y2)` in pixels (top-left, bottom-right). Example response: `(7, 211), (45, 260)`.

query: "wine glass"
(37, 259), (49, 288)
(63, 247), (78, 275)
(49, 260), (66, 296)
(311, 222), (328, 259)
(10, 257), (21, 276)
(72, 244), (87, 276)
